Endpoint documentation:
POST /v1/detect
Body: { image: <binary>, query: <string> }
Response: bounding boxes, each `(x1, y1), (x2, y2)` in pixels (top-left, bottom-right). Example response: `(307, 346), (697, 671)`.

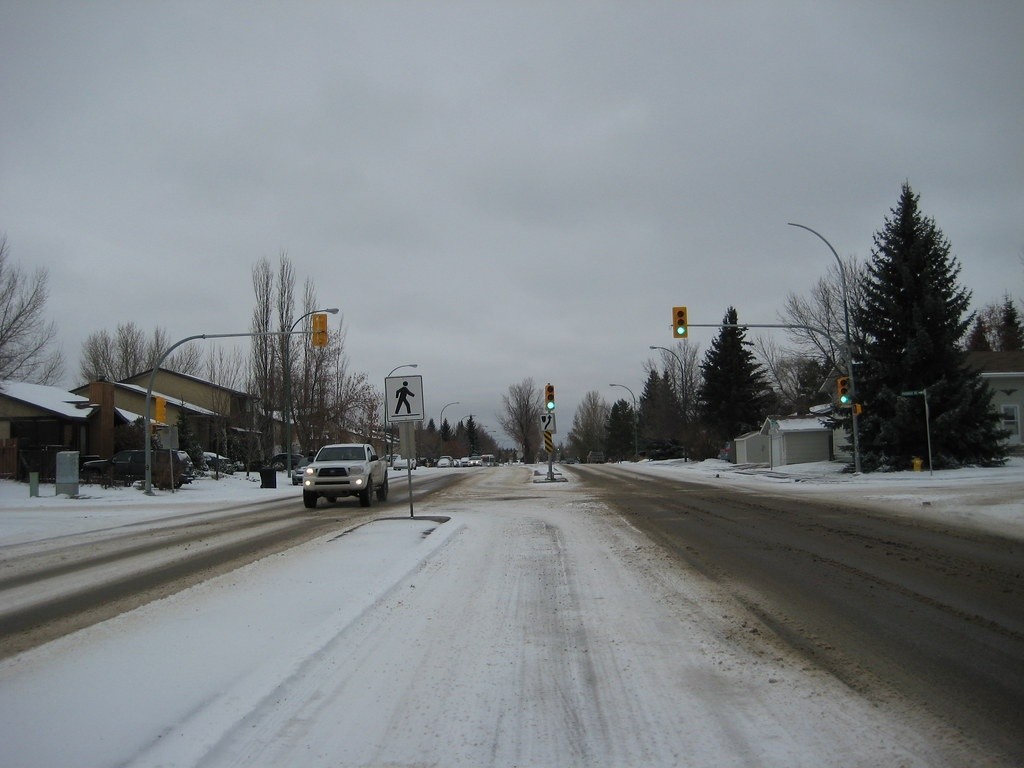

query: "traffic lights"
(673, 306), (688, 338)
(852, 404), (862, 417)
(545, 385), (555, 411)
(839, 377), (848, 405)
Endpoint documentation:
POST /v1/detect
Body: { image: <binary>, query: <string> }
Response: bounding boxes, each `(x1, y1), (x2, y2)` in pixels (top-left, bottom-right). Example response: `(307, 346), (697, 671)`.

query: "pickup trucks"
(301, 443), (388, 508)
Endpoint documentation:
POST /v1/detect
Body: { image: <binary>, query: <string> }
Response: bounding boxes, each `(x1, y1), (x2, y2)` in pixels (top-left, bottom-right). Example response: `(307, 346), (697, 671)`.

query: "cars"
(292, 457), (317, 485)
(635, 451), (652, 458)
(385, 455), (400, 467)
(392, 456), (417, 470)
(437, 458), (451, 467)
(251, 453), (304, 472)
(719, 441), (735, 463)
(191, 452), (232, 472)
(453, 456), (483, 467)
(568, 457), (575, 463)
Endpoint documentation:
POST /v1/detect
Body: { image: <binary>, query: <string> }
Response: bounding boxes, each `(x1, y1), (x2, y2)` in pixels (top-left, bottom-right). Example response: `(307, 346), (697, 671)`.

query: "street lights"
(383, 363), (417, 456)
(440, 401), (459, 456)
(461, 414), (476, 456)
(285, 308), (340, 477)
(610, 383), (638, 456)
(787, 222), (861, 474)
(650, 345), (688, 463)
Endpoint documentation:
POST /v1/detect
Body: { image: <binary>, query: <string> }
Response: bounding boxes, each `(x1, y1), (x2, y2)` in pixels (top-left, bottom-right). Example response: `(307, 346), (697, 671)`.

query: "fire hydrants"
(912, 458), (923, 472)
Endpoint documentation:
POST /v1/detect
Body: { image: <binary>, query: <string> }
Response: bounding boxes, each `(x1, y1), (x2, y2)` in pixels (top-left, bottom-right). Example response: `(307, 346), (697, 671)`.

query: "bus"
(481, 455), (495, 466)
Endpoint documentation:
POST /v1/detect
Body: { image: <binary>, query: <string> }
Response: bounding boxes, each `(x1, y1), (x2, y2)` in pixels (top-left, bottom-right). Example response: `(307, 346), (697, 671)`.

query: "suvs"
(587, 451), (605, 464)
(440, 456), (454, 467)
(80, 449), (196, 490)
